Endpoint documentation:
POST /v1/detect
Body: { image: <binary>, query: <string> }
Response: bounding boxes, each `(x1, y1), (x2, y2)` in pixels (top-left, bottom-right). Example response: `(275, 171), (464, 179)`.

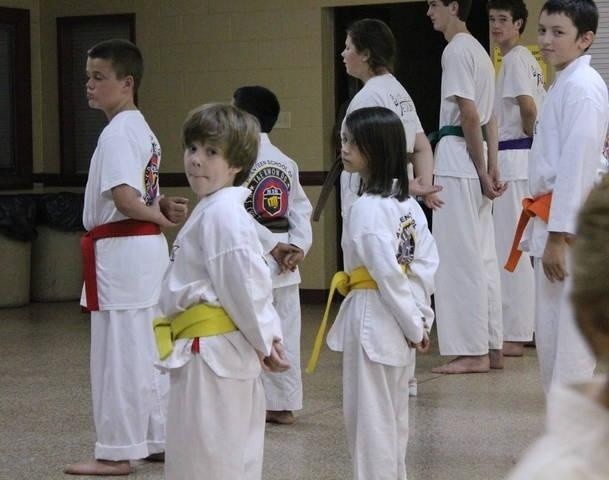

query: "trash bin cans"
(0, 192), (86, 309)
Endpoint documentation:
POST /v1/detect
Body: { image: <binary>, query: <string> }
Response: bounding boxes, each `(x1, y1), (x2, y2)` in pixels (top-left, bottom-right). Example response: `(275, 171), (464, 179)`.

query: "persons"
(150, 100), (290, 479)
(223, 79), (314, 428)
(501, 172), (609, 480)
(306, 103), (436, 480)
(62, 36), (190, 478)
(484, 0), (547, 359)
(502, 0), (608, 396)
(424, 1), (512, 377)
(311, 15), (447, 398)
